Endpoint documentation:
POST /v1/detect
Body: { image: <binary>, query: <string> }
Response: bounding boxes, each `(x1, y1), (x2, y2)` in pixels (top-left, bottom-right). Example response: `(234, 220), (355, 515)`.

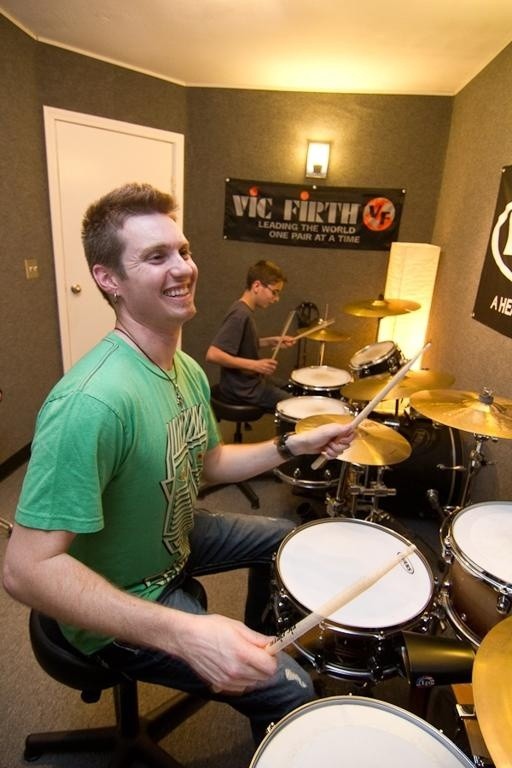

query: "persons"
(205, 260), (299, 411)
(3, 181), (359, 695)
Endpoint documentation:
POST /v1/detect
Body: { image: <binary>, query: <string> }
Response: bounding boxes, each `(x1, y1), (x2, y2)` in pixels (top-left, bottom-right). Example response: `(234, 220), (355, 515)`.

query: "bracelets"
(270, 432), (298, 464)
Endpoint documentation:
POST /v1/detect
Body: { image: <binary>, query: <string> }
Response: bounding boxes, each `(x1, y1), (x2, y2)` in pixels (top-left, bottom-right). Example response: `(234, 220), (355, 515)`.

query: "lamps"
(375, 242), (442, 372)
(304, 139), (333, 183)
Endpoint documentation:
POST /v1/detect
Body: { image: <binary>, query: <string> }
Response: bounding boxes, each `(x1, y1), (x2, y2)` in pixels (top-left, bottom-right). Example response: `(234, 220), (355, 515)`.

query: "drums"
(439, 500), (511, 651)
(360, 410), (462, 525)
(270, 517), (434, 685)
(350, 341), (405, 379)
(249, 695), (475, 767)
(274, 396), (355, 489)
(288, 365), (351, 402)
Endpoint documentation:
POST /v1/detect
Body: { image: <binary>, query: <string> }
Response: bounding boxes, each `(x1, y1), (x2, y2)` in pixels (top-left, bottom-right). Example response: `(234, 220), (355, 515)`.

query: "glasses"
(262, 280), (279, 296)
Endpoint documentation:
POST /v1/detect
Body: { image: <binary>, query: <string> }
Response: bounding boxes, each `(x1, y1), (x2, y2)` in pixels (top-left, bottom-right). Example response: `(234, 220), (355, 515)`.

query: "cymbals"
(340, 368), (456, 416)
(471, 615), (511, 767)
(401, 388), (511, 440)
(342, 299), (421, 317)
(295, 415), (413, 467)
(296, 327), (351, 341)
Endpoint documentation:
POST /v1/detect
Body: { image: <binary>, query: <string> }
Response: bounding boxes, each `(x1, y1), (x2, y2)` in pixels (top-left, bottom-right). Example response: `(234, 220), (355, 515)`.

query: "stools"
(23, 578), (206, 768)
(197, 385), (282, 509)
